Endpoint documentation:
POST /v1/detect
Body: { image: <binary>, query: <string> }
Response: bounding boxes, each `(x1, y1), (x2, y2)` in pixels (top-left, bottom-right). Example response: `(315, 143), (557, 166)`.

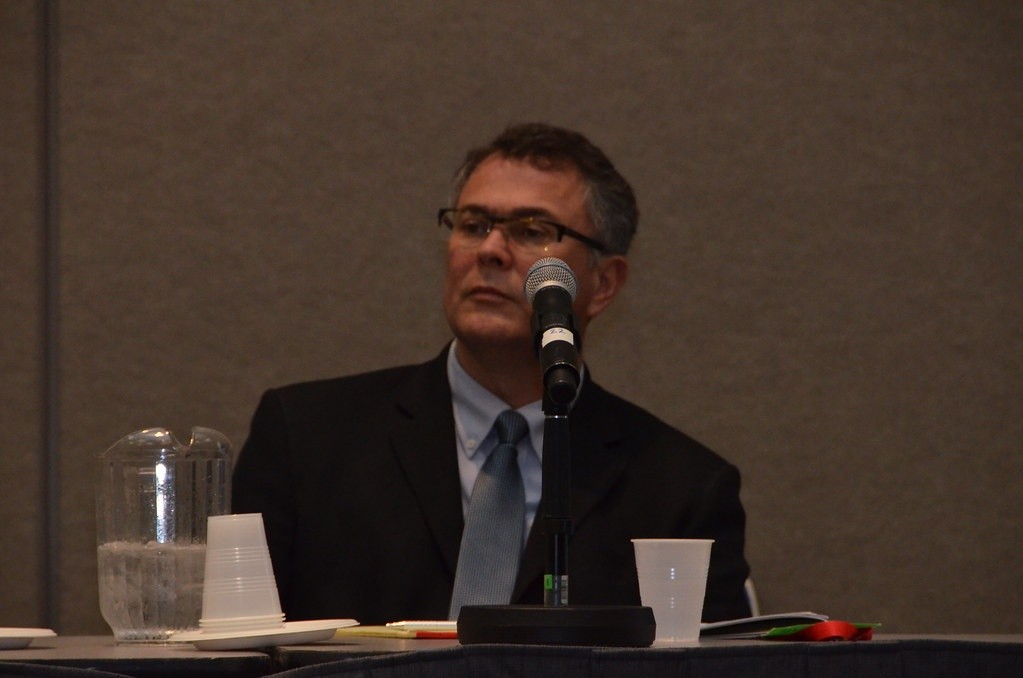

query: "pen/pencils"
(385, 619), (457, 632)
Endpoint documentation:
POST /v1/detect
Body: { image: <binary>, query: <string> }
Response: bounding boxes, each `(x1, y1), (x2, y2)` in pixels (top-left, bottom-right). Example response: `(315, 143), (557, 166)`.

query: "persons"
(232, 123), (750, 640)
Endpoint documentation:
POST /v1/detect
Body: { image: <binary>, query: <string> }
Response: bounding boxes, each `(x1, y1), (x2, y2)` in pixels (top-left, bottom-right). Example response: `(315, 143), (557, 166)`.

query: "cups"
(95, 427), (233, 641)
(198, 512), (285, 634)
(631, 538), (714, 643)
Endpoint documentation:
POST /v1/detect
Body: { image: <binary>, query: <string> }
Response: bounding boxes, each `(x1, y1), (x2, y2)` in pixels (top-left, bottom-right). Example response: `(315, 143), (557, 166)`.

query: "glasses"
(436, 208), (604, 255)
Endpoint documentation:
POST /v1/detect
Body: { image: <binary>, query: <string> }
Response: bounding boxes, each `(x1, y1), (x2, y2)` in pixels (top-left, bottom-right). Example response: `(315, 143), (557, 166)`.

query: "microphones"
(524, 256), (581, 407)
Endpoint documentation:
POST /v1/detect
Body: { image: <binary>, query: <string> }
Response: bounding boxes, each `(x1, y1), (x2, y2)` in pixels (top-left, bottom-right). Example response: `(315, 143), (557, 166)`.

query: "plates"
(166, 620), (361, 652)
(0, 627), (59, 649)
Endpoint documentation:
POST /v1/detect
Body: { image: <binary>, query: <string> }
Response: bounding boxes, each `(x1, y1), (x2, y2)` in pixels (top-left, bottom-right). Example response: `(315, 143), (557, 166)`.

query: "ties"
(448, 410), (530, 621)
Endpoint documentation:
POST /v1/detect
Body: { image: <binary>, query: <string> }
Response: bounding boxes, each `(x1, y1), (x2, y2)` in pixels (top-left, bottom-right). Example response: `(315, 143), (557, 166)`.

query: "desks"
(0, 626), (1023, 678)
